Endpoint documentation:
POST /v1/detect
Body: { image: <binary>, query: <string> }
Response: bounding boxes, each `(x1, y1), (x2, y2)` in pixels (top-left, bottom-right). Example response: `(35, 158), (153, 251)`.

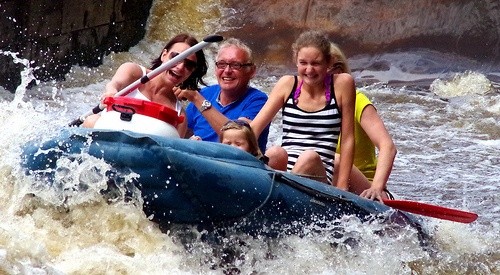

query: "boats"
(20, 129), (435, 273)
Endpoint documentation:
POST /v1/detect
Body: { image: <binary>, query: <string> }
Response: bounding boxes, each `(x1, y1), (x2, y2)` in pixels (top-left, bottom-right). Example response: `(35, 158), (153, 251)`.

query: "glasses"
(221, 120), (251, 130)
(215, 60), (252, 70)
(168, 50), (197, 71)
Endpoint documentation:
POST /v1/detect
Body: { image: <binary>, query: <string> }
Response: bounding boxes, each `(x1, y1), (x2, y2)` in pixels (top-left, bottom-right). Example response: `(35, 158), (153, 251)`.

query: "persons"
(250, 32), (355, 191)
(82, 34), (212, 138)
(327, 44), (396, 205)
(218, 120), (259, 161)
(172, 38), (272, 155)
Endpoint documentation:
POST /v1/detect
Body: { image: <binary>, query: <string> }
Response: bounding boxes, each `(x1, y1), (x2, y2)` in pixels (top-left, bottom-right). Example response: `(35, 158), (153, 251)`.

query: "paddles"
(383, 199), (477, 225)
(60, 35), (223, 129)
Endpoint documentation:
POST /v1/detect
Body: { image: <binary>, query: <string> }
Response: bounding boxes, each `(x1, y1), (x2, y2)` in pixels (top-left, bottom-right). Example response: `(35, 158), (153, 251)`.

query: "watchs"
(198, 99), (212, 112)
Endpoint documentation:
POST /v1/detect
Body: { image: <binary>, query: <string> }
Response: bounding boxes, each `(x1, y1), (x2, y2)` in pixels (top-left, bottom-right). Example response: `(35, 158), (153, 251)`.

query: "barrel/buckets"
(92, 96), (184, 138)
